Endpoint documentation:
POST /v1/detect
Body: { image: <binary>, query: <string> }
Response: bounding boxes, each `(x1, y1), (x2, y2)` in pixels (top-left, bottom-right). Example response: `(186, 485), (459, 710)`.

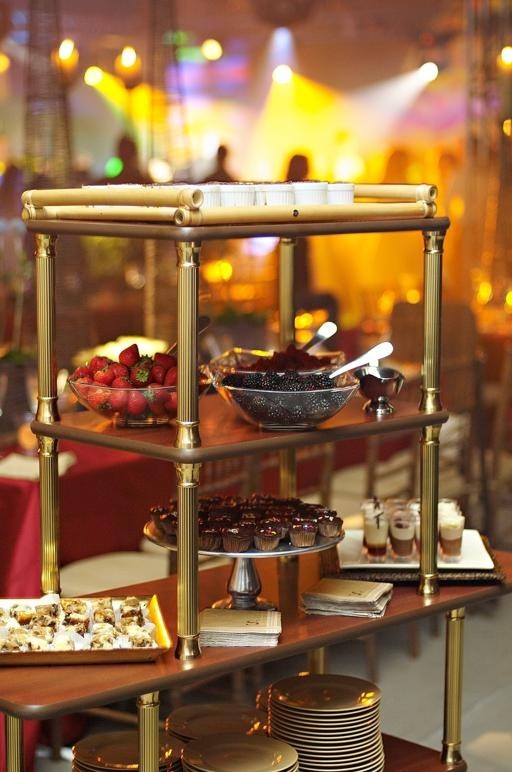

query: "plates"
(70, 670), (385, 772)
(334, 527), (495, 571)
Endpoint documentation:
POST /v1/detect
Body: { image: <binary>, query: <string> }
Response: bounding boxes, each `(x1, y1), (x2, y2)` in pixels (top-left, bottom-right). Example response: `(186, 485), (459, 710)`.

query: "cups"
(352, 366), (406, 418)
(360, 497), (464, 560)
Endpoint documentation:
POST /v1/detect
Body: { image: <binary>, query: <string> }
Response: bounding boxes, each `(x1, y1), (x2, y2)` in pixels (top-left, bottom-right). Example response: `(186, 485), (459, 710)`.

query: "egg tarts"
(150, 494), (343, 553)
(0, 596), (153, 651)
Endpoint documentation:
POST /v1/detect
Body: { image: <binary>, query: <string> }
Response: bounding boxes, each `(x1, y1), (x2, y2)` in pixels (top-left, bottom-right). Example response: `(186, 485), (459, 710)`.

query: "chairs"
(365, 300), (494, 537)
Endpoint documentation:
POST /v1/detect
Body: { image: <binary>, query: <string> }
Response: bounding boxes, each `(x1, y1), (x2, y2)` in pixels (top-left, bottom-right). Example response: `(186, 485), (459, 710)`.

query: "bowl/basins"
(66, 376), (213, 427)
(205, 345), (360, 435)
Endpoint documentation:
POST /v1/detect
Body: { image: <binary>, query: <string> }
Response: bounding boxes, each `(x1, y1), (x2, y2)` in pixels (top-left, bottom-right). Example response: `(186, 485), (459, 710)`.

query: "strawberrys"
(73, 344), (176, 418)
(242, 344), (331, 370)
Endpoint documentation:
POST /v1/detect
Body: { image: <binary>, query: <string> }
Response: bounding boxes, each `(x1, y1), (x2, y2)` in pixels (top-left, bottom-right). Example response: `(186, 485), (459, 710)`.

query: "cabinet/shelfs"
(1, 181), (512, 771)
